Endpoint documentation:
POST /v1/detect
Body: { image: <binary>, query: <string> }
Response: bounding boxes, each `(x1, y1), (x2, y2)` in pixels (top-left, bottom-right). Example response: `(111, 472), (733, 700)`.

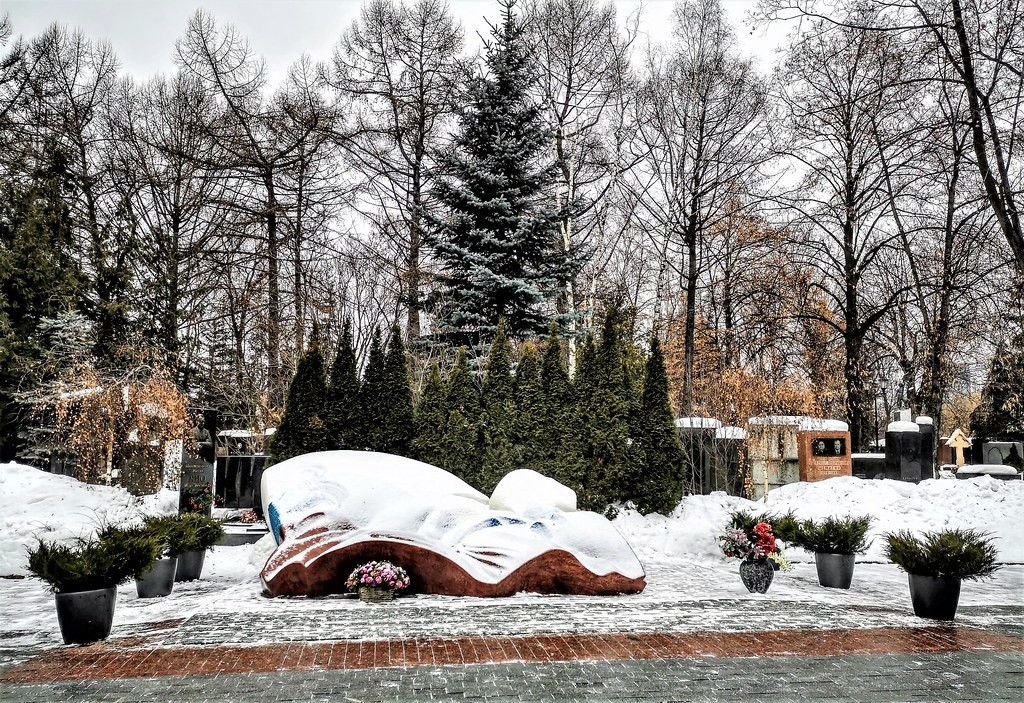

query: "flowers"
(344, 560), (410, 593)
(187, 488), (225, 511)
(719, 522), (777, 559)
(241, 511), (258, 523)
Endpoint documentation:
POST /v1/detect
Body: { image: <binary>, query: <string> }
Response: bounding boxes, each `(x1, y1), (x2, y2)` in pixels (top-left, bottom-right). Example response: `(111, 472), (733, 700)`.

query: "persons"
(816, 440), (830, 456)
(186, 414), (213, 460)
(831, 440), (846, 456)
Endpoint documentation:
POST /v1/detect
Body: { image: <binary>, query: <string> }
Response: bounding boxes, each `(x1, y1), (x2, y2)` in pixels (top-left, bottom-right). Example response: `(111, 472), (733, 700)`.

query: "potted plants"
(96, 516), (200, 599)
(143, 511), (226, 584)
(876, 525), (1002, 622)
(19, 532), (157, 647)
(777, 512), (880, 589)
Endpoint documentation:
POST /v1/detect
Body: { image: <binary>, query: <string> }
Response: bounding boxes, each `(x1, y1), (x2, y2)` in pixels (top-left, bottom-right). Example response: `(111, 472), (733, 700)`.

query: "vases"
(358, 585), (395, 603)
(739, 555), (774, 594)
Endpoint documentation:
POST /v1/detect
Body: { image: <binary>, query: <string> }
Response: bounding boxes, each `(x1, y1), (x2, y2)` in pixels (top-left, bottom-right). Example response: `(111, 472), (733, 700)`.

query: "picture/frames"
(812, 437), (846, 457)
